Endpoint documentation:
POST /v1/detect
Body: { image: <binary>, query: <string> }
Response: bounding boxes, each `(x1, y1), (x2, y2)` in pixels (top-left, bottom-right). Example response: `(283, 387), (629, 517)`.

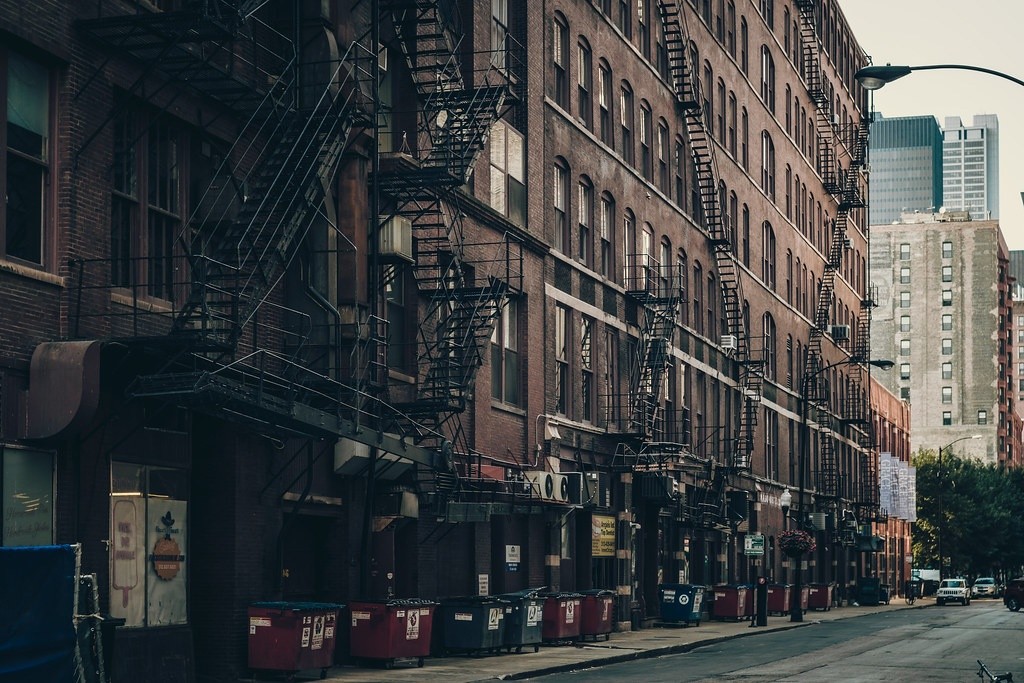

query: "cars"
(972, 577), (1000, 599)
(1004, 579), (1024, 611)
(936, 578), (970, 605)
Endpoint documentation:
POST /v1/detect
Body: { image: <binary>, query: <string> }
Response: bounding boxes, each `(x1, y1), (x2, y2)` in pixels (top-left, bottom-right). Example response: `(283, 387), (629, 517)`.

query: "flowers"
(776, 528), (818, 559)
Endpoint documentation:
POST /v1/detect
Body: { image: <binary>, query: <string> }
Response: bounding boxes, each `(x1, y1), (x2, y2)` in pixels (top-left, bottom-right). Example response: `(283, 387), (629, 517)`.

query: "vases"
(785, 547), (811, 559)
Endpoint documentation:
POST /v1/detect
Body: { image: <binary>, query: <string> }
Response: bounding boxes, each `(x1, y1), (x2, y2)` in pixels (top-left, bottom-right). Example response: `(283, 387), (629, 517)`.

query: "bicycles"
(904, 581), (918, 605)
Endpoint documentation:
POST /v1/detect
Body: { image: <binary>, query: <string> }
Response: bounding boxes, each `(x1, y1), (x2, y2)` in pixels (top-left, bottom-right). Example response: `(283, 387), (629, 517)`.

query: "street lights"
(791, 360), (895, 619)
(779, 485), (791, 582)
(938, 433), (984, 575)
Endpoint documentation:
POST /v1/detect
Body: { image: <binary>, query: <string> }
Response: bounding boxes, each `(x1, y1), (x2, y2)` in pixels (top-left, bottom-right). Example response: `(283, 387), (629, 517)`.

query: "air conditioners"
(554, 473), (570, 502)
(830, 114), (839, 125)
(832, 324), (851, 341)
(861, 524), (870, 536)
(843, 238), (854, 249)
(522, 470), (554, 500)
(376, 214), (412, 257)
(720, 334), (738, 350)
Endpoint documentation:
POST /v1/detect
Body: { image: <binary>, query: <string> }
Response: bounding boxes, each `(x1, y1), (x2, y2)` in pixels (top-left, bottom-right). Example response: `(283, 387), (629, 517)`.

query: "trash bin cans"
(581, 588), (616, 643)
(539, 591), (588, 645)
(348, 599), (435, 670)
(658, 582), (706, 630)
(905, 580), (925, 601)
(711, 582), (747, 622)
(767, 584), (810, 616)
(494, 586), (548, 653)
(246, 600), (346, 680)
(879, 583), (892, 607)
(808, 582), (835, 611)
(437, 593), (513, 656)
